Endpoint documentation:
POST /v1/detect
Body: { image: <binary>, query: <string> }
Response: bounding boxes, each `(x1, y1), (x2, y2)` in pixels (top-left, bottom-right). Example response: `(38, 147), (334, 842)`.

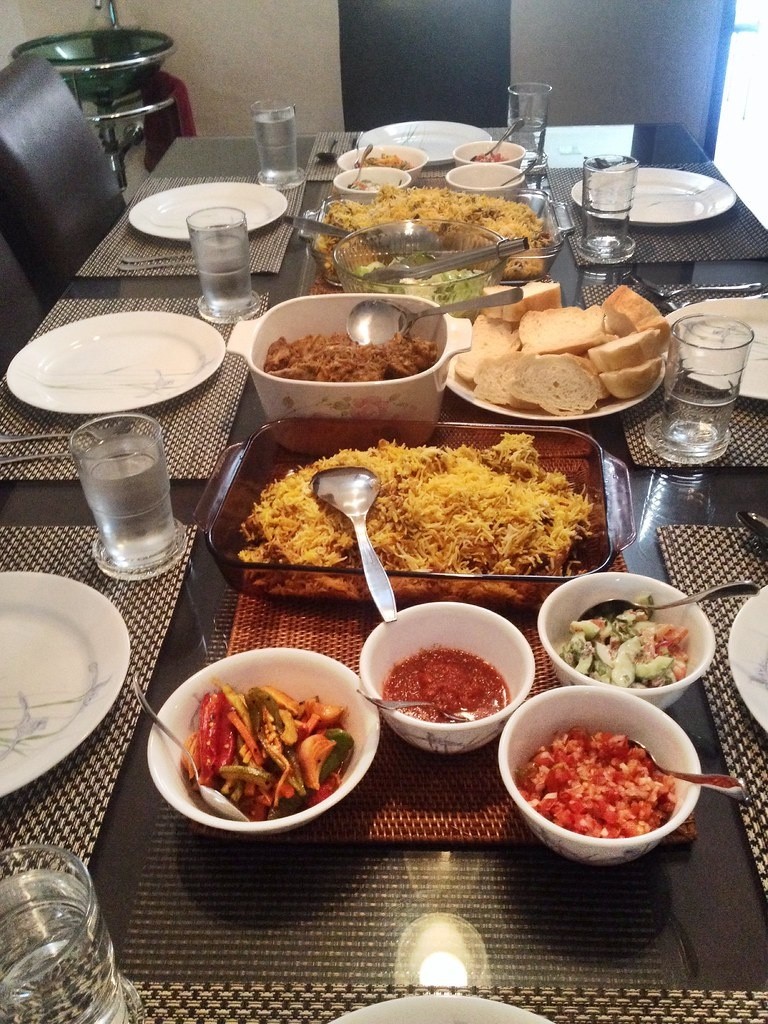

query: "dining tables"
(0, 122), (768, 1024)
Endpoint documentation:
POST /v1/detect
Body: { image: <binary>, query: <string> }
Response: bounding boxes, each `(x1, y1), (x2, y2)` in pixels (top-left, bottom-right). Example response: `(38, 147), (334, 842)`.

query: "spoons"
(345, 287), (530, 348)
(576, 582), (760, 619)
(627, 738), (747, 799)
(130, 668), (251, 822)
(316, 139), (339, 162)
(357, 689), (477, 721)
(734, 508), (768, 540)
(310, 467), (398, 622)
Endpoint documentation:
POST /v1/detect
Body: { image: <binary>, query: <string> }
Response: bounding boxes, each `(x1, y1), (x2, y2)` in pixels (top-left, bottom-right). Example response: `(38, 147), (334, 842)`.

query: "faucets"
(95, 0), (119, 30)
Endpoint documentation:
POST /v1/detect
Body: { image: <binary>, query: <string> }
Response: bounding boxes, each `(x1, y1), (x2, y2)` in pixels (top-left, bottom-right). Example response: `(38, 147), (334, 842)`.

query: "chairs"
(0, 53), (125, 316)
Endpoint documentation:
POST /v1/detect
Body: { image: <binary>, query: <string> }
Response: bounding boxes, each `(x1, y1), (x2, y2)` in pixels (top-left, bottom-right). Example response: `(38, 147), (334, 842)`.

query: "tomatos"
(515, 729), (674, 840)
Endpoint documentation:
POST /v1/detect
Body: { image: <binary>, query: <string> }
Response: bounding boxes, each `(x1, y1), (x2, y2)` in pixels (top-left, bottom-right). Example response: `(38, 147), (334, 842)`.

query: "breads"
(453, 281), (671, 416)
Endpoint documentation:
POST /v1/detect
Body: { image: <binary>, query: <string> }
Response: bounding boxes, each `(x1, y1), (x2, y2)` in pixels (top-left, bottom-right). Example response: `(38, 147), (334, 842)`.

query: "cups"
(0, 847), (130, 1024)
(69, 101), (297, 568)
(580, 155), (754, 448)
(508, 82), (552, 158)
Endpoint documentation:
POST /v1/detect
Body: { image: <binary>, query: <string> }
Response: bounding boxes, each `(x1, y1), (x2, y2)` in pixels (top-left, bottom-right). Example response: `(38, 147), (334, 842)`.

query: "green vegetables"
(353, 252), (487, 322)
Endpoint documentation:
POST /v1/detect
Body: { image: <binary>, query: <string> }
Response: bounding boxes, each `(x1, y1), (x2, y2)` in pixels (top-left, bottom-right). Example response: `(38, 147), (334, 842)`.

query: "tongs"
(363, 238), (528, 281)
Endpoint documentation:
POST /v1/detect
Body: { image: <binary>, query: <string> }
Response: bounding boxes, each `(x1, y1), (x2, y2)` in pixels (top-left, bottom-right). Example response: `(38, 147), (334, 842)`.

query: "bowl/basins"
(227, 293), (473, 456)
(334, 141), (527, 205)
(334, 218), (505, 305)
(145, 571), (717, 863)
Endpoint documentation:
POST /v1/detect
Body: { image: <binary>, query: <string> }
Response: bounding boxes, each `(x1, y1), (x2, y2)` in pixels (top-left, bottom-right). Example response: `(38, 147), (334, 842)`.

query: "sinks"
(10, 27), (178, 103)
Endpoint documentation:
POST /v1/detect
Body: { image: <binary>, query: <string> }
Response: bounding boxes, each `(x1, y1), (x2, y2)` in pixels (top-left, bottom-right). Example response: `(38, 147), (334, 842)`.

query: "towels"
(142, 71), (196, 174)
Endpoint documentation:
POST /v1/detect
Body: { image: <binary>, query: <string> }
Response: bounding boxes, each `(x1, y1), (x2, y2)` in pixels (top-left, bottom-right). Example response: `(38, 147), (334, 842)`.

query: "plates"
(321, 994), (550, 1024)
(128, 181), (288, 239)
(446, 354), (666, 420)
(359, 120), (492, 167)
(5, 312), (226, 414)
(729, 583), (768, 732)
(571, 167), (735, 226)
(0, 571), (131, 795)
(661, 300), (767, 399)
